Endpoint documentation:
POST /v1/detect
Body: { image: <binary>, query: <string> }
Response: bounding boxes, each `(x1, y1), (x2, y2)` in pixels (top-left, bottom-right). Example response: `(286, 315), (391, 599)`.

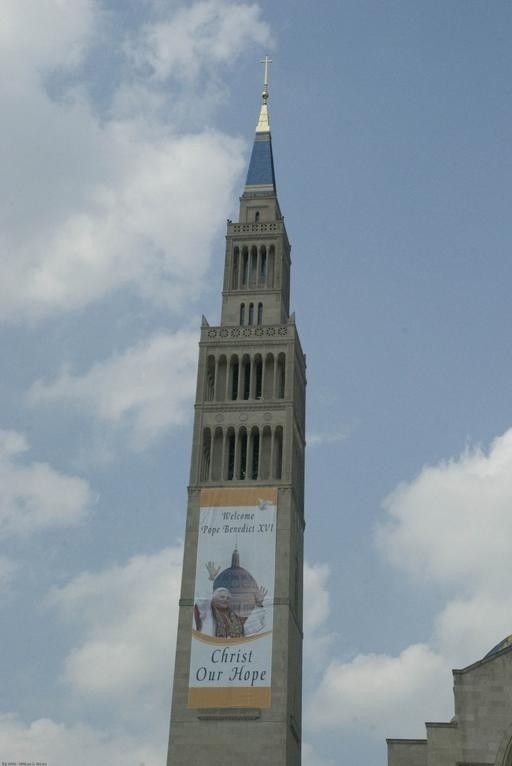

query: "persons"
(194, 561), (268, 639)
